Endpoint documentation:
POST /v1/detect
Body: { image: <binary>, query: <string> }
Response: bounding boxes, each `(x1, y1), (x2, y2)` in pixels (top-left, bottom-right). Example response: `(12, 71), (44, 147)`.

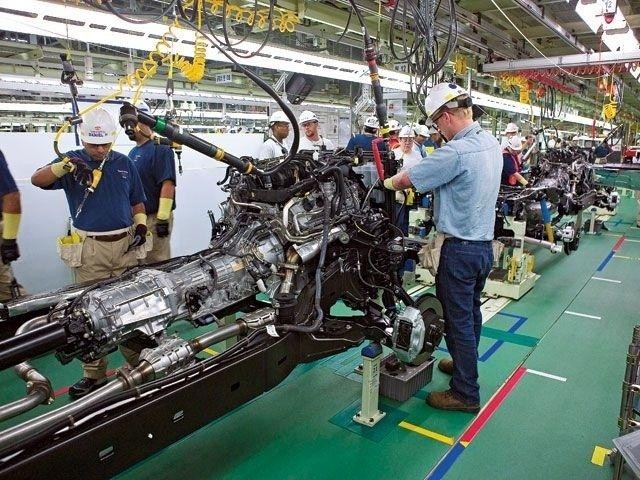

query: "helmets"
(505, 122), (519, 133)
(424, 82), (469, 119)
(387, 119), (404, 132)
(398, 125), (417, 139)
(508, 136), (524, 150)
(76, 105), (118, 145)
(364, 115), (381, 130)
(298, 109), (320, 124)
(267, 109), (291, 124)
(126, 97), (152, 120)
(415, 123), (431, 139)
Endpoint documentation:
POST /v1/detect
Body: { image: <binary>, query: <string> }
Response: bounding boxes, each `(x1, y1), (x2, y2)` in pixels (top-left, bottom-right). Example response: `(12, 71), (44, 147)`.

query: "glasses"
(432, 111), (455, 125)
(301, 120), (319, 128)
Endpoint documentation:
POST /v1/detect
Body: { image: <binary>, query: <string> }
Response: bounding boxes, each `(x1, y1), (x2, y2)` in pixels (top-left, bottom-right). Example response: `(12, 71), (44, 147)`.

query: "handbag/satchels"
(54, 232), (85, 270)
(416, 227), (445, 276)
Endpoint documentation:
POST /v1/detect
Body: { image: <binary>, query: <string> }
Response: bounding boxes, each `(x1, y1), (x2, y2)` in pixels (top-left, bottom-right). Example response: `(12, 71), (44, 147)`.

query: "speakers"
(286, 72), (315, 105)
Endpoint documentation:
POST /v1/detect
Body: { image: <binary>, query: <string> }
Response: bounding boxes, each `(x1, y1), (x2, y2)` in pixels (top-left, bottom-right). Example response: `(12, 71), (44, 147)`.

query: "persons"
(297, 110), (335, 154)
(258, 111), (290, 162)
(0, 150), (32, 302)
(385, 81), (504, 413)
(346, 116), (386, 151)
(383, 119), (561, 224)
(118, 103), (177, 266)
(572, 136), (579, 145)
(30, 106), (147, 398)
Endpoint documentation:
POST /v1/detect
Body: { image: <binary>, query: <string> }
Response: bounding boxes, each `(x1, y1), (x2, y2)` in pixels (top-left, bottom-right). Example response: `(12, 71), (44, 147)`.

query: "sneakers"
(425, 388), (480, 416)
(438, 357), (458, 376)
(68, 374), (108, 398)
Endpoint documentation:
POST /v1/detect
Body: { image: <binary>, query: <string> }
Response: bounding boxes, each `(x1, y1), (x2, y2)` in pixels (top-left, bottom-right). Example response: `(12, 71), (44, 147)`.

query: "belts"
(86, 232), (128, 243)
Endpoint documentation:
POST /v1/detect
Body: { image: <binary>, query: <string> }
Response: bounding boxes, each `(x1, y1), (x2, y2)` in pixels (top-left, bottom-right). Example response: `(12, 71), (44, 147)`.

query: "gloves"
(0, 239), (21, 264)
(128, 223), (149, 248)
(70, 159), (96, 185)
(154, 217), (171, 239)
